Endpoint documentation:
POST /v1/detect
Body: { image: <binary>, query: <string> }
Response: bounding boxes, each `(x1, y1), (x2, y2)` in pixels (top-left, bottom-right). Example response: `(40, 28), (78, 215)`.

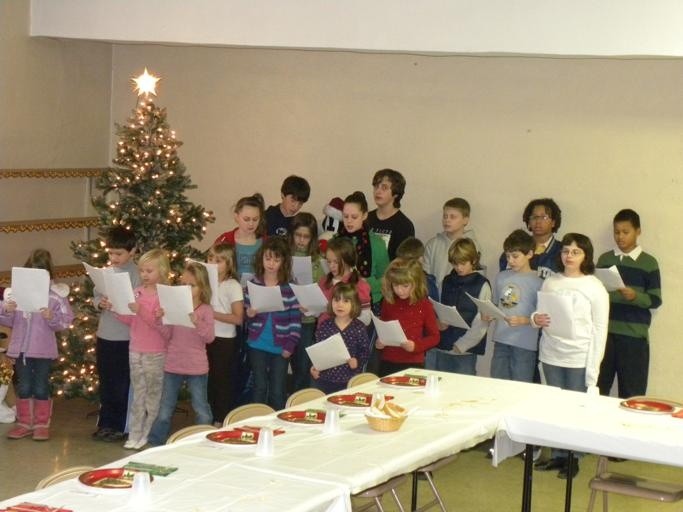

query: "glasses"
(531, 214), (550, 221)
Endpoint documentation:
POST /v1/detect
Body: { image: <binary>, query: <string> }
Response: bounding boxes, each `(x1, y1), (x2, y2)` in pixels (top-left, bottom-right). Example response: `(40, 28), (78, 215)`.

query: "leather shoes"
(533, 457), (565, 471)
(558, 458), (579, 479)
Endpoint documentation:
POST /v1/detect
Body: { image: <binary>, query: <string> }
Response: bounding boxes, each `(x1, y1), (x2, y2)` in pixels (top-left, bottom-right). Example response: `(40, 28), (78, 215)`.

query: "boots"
(8, 398), (33, 439)
(33, 399), (54, 439)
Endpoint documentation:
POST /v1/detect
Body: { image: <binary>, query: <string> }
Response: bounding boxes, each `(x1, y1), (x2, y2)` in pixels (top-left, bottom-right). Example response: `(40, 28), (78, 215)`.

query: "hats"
(323, 197), (344, 223)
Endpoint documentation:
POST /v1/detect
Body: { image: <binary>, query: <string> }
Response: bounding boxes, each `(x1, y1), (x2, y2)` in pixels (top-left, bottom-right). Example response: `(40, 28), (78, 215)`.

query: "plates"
(206, 429), (259, 445)
(277, 410), (326, 425)
(618, 394), (683, 415)
(380, 374), (427, 387)
(76, 466), (153, 492)
(326, 394), (371, 408)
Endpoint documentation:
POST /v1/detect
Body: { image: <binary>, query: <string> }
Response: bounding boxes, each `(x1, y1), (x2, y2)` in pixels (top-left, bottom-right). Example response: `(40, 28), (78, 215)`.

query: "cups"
(257, 427), (284, 463)
(323, 406), (342, 434)
(426, 373), (439, 398)
(581, 383), (601, 411)
(127, 470), (156, 504)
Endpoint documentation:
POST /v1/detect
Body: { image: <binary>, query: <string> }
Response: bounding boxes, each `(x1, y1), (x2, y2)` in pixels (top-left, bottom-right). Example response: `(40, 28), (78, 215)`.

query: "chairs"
(587, 396), (683, 512)
(223, 404), (275, 426)
(35, 465), (95, 490)
(286, 388), (408, 512)
(347, 373), (457, 512)
(167, 424), (216, 445)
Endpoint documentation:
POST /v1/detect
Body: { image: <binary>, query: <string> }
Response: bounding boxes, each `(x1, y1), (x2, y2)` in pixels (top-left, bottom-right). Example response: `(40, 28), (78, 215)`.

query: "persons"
(596, 208), (663, 400)
(330, 191), (390, 352)
(141, 262), (215, 449)
(265, 175), (310, 240)
(530, 233), (609, 393)
(106, 248), (169, 450)
(92, 228), (137, 442)
(499, 198), (565, 273)
(481, 230), (545, 380)
(0, 248), (73, 441)
(316, 236), (371, 329)
(309, 283), (369, 394)
(213, 194), (268, 405)
(422, 198), (486, 303)
(362, 171), (415, 262)
(374, 257), (440, 378)
(241, 240), (301, 410)
(434, 238), (491, 374)
(395, 236), (425, 262)
(205, 243), (244, 428)
(285, 213), (325, 392)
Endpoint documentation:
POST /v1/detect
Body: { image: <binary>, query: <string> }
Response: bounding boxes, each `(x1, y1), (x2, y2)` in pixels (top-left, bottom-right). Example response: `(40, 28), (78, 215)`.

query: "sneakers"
(92, 428), (147, 450)
